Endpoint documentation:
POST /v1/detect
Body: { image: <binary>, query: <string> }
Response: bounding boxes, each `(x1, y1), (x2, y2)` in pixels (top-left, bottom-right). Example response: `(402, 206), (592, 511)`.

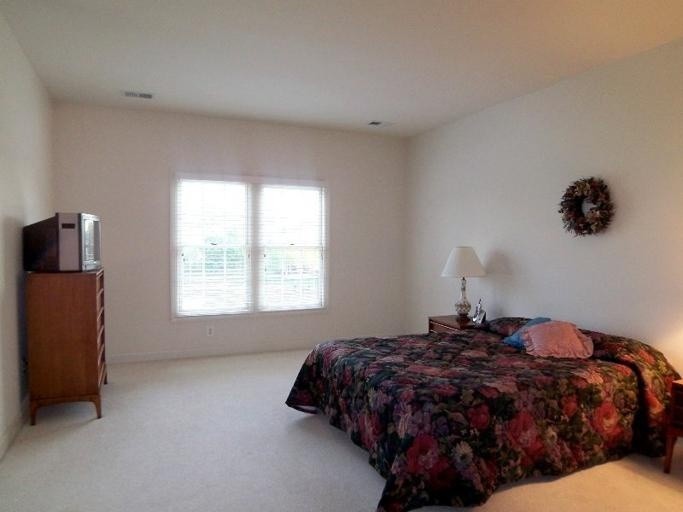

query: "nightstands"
(428, 315), (475, 334)
(661, 379), (682, 472)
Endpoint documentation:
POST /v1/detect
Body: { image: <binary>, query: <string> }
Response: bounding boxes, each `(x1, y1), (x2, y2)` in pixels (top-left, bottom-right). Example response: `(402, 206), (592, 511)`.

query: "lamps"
(440, 247), (487, 318)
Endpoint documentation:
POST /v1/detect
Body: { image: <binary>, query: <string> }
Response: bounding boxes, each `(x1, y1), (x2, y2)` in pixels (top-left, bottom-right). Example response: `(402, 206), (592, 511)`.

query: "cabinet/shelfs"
(24, 269), (109, 423)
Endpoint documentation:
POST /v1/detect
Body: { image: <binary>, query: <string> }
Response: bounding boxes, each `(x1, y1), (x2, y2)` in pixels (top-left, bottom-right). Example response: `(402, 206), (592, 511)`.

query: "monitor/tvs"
(22, 212), (103, 272)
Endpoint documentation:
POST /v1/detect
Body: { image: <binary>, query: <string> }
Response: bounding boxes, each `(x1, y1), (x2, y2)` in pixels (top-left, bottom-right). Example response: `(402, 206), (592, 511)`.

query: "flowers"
(556, 176), (615, 240)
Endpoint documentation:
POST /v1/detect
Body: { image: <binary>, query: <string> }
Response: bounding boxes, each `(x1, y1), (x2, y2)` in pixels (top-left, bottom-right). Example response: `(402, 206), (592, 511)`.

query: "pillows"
(504, 316), (589, 361)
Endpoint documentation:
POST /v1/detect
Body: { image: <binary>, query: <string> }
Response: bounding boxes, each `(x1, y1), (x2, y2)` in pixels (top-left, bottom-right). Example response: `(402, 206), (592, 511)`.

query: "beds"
(311, 317), (671, 512)
